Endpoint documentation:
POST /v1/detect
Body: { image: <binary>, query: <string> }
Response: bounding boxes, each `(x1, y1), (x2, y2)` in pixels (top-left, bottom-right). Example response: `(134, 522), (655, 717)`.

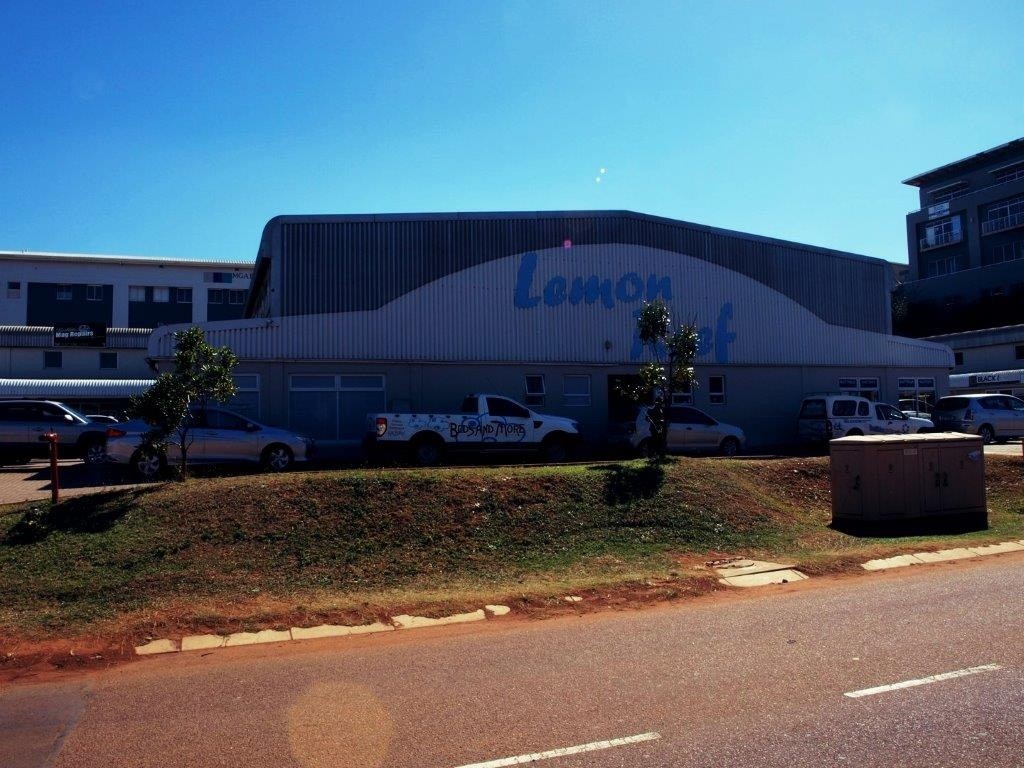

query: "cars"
(931, 393), (1023, 446)
(105, 406), (314, 481)
(0, 399), (109, 467)
(899, 399), (935, 419)
(629, 404), (746, 458)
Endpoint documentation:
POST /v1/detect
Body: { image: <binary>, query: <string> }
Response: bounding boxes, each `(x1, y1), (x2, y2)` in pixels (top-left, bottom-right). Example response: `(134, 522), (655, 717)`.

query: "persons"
(635, 395), (666, 459)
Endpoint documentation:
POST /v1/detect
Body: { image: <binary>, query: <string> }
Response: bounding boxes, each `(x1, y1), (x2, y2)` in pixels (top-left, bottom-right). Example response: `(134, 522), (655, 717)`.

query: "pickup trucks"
(365, 392), (581, 468)
(797, 391), (936, 444)
